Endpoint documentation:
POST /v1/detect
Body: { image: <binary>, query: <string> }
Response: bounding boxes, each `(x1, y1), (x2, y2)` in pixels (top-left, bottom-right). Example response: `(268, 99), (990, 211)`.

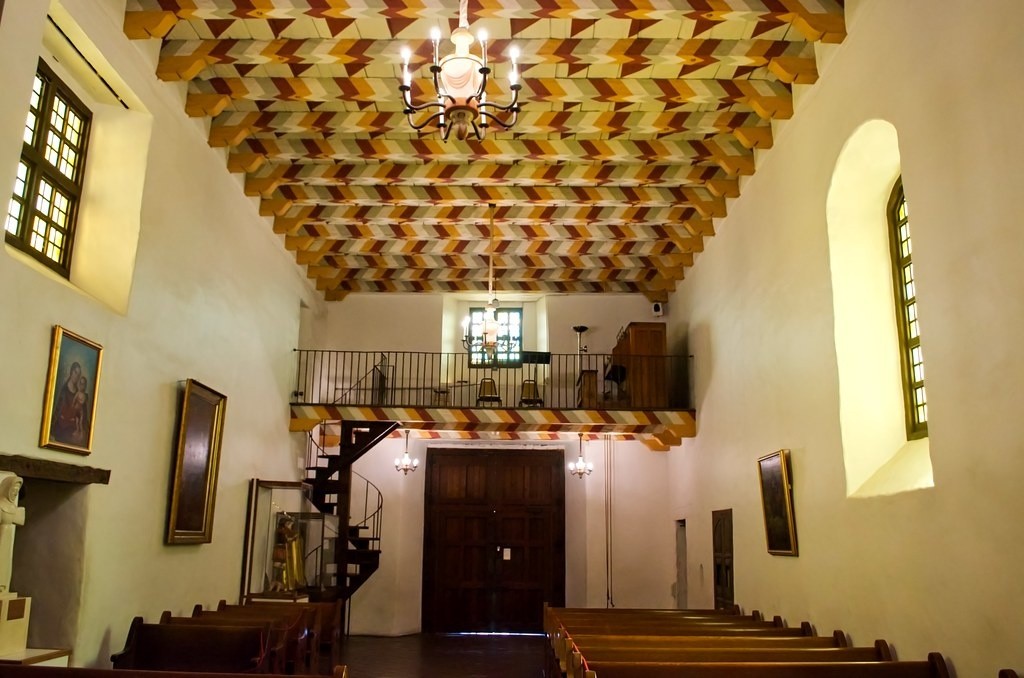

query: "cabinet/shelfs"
(575, 368), (597, 410)
(608, 319), (669, 408)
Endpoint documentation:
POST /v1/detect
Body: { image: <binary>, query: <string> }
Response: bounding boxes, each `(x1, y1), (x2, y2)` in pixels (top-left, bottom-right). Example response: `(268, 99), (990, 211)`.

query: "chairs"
(518, 378), (543, 407)
(472, 377), (502, 408)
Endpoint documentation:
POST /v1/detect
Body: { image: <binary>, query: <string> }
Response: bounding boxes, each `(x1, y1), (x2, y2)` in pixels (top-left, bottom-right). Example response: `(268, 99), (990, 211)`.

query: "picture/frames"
(39, 324), (104, 455)
(162, 377), (229, 544)
(755, 448), (797, 556)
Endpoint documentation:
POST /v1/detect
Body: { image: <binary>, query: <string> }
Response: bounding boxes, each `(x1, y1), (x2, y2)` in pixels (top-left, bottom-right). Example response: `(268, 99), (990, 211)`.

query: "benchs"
(109, 595), (334, 676)
(541, 603), (950, 677)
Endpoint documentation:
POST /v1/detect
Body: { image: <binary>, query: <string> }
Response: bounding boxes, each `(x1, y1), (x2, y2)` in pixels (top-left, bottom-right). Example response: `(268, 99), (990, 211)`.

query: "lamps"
(394, 429), (419, 473)
(567, 432), (593, 479)
(397, 0), (521, 140)
(460, 203), (520, 360)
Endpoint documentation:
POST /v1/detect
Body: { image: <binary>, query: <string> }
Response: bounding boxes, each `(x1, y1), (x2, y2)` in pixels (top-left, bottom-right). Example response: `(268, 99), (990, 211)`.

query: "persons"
(0, 477), (25, 593)
(270, 517), (297, 593)
